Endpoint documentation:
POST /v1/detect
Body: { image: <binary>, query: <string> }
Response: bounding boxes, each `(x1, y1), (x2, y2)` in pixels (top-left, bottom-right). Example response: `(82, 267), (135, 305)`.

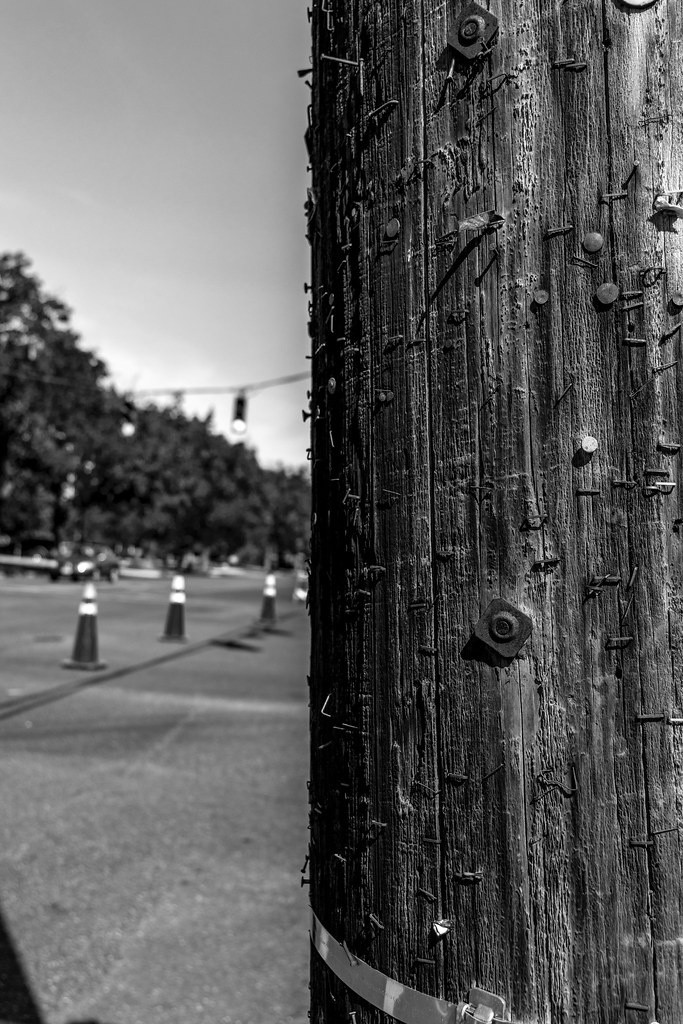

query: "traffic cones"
(259, 574), (278, 624)
(158, 574), (189, 644)
(62, 583), (109, 670)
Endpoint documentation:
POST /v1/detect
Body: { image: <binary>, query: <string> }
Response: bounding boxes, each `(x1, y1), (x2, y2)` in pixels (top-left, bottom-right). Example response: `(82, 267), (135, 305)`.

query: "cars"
(50, 543), (121, 583)
